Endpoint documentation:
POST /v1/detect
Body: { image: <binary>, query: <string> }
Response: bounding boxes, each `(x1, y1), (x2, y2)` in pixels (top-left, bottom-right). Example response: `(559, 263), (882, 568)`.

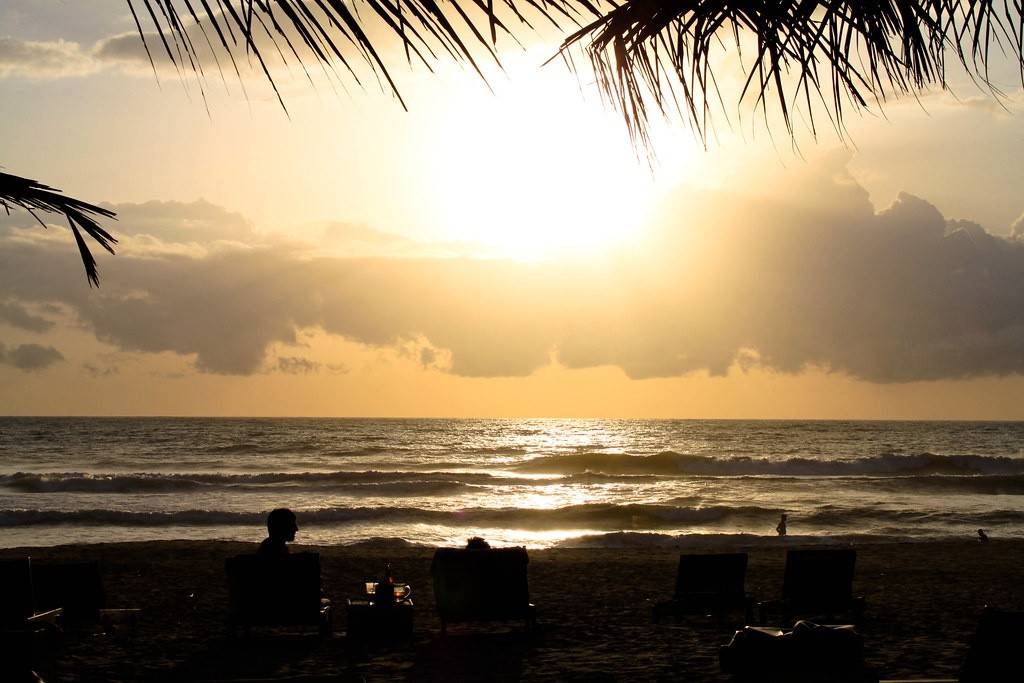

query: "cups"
(366, 582), (379, 605)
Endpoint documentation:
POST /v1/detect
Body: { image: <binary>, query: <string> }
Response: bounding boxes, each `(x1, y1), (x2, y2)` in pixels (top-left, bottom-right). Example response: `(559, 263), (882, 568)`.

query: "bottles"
(383, 563), (397, 603)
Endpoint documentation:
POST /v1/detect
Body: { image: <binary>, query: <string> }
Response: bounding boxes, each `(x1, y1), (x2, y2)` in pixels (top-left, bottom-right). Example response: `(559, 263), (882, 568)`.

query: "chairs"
(429, 546), (541, 638)
(758, 547), (864, 628)
(225, 553), (332, 651)
(650, 551), (754, 630)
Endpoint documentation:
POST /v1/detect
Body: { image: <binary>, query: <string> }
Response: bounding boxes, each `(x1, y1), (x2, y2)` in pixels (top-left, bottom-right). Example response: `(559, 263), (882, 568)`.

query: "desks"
(345, 598), (417, 644)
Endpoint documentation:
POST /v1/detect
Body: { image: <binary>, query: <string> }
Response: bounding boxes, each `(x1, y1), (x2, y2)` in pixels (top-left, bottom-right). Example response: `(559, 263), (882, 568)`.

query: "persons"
(777, 514), (787, 536)
(256, 508), (298, 556)
(977, 529), (988, 542)
(465, 537), (491, 549)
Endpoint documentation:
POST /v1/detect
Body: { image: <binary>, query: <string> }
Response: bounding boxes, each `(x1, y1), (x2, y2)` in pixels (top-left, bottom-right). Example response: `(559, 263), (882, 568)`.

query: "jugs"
(392, 583), (411, 603)
